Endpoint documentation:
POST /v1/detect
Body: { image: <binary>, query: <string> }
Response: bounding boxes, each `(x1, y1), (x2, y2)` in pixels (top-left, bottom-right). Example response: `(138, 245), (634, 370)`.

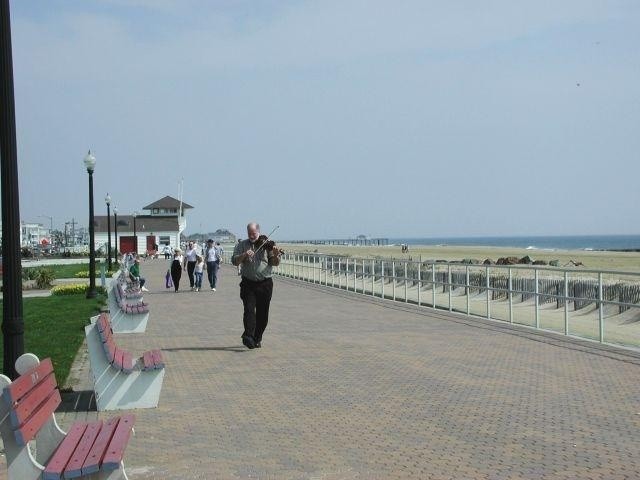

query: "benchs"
(106, 270), (150, 333)
(0, 311), (165, 480)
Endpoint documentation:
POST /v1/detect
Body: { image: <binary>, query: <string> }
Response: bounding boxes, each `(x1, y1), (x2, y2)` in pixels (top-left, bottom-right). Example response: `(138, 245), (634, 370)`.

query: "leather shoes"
(243, 336), (260, 349)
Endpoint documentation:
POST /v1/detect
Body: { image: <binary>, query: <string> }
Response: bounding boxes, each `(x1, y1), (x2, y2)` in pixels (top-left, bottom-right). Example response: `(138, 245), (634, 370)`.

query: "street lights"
(134, 211), (136, 253)
(105, 193), (112, 272)
(83, 149), (98, 298)
(113, 206), (118, 262)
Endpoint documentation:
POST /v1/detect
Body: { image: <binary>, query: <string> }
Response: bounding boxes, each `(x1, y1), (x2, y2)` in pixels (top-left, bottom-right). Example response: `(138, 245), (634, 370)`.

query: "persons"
(192, 257), (207, 293)
(179, 237), (224, 264)
(204, 239), (223, 293)
(169, 248), (174, 257)
(161, 242), (170, 261)
(183, 241), (200, 288)
(230, 222), (282, 350)
(170, 247), (184, 294)
(237, 238), (245, 276)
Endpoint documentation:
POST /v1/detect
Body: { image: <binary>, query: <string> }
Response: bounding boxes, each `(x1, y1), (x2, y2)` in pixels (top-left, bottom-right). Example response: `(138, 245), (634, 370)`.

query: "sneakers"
(211, 288), (216, 292)
(141, 286), (149, 291)
(191, 286), (201, 292)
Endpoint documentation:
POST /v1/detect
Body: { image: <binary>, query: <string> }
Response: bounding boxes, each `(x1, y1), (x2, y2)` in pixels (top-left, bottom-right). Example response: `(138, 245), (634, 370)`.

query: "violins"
(254, 235), (285, 254)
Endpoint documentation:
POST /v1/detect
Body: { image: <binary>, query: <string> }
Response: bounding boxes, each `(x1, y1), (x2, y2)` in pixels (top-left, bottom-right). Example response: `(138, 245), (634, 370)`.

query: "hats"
(206, 239), (214, 242)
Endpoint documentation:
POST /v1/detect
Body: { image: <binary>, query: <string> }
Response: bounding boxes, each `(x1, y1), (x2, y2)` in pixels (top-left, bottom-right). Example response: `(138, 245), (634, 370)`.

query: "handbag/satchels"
(166, 272), (173, 287)
